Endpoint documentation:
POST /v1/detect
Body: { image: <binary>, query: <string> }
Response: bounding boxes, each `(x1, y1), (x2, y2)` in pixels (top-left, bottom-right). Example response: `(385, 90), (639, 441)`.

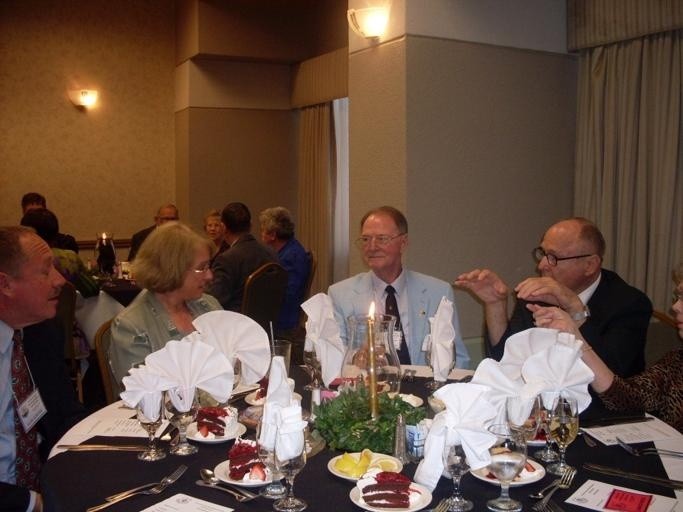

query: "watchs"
(568, 304), (591, 320)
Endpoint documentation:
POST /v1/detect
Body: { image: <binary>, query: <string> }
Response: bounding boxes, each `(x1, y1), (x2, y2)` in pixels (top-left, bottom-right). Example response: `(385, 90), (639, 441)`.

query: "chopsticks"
(584, 463), (683, 493)
(58, 444), (155, 451)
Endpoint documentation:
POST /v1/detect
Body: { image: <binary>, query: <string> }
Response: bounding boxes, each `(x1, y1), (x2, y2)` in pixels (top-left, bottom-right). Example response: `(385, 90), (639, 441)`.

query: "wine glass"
(547, 398), (579, 476)
(486, 425), (527, 512)
(164, 388), (200, 456)
(541, 392), (559, 420)
(256, 416), (288, 498)
(426, 336), (456, 390)
(443, 439), (474, 512)
(232, 359), (241, 390)
(536, 390), (569, 462)
(274, 426), (308, 511)
(303, 345), (327, 391)
(136, 393), (168, 461)
(506, 397), (541, 460)
(506, 398), (534, 428)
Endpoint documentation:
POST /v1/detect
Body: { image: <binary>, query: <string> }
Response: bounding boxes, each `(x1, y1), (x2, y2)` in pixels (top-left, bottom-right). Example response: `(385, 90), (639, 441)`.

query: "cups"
(120, 262), (131, 278)
(265, 340), (291, 378)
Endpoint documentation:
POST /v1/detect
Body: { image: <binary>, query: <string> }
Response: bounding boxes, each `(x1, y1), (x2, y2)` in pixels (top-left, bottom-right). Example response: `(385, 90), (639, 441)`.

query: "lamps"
(68, 88), (99, 109)
(347, 6), (391, 44)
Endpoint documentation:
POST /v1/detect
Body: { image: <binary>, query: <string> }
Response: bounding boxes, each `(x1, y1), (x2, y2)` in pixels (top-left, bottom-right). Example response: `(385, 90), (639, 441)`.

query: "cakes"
(197, 406), (238, 438)
(359, 471), (410, 508)
(226, 441), (265, 480)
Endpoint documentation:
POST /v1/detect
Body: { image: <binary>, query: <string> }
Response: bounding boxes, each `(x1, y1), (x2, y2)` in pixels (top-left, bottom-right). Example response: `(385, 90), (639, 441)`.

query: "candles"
(365, 302), (379, 424)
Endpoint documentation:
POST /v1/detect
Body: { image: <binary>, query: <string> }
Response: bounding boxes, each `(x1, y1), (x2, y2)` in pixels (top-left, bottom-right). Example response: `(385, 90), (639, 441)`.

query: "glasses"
(187, 264), (211, 276)
(355, 232), (404, 246)
(533, 247), (592, 267)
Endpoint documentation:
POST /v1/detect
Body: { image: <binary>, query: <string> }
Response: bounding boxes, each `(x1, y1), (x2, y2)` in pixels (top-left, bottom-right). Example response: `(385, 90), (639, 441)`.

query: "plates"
(328, 452), (404, 484)
(351, 481), (433, 511)
(471, 458), (546, 488)
(335, 364), (367, 382)
(231, 383), (260, 396)
(245, 390), (302, 406)
(185, 421), (248, 444)
(398, 393), (424, 408)
(525, 438), (549, 447)
(214, 460), (286, 488)
(361, 382), (390, 394)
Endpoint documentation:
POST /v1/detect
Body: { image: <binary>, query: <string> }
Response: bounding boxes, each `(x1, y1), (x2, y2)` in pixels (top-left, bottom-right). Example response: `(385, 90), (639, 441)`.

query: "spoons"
(577, 430), (596, 448)
(529, 479), (560, 499)
(200, 468), (261, 501)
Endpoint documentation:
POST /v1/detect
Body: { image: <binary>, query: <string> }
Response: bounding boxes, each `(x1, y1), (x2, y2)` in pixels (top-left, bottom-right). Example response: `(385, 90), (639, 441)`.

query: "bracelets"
(580, 345), (593, 353)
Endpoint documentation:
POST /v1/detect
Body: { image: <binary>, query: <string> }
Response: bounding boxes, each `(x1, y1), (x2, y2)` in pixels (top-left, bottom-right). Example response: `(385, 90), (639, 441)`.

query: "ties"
(12, 330), (44, 496)
(385, 285), (411, 366)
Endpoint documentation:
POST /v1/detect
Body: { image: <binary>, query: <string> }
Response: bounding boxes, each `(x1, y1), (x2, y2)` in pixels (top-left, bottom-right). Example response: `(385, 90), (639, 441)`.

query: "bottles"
(392, 410), (414, 464)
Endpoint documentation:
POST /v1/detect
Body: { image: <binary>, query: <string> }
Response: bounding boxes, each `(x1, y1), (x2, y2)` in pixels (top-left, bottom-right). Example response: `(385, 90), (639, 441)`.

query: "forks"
(104, 464), (187, 501)
(195, 479), (268, 503)
(616, 437), (683, 461)
(400, 369), (410, 383)
(531, 467), (576, 511)
(408, 371), (416, 382)
(86, 477), (171, 512)
(420, 497), (451, 512)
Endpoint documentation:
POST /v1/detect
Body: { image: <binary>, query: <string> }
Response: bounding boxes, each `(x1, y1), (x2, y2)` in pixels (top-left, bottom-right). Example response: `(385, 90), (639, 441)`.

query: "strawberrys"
(251, 466), (263, 479)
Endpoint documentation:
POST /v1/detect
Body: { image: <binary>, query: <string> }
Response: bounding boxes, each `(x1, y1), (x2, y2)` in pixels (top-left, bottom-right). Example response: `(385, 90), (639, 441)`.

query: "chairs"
(642, 309), (679, 368)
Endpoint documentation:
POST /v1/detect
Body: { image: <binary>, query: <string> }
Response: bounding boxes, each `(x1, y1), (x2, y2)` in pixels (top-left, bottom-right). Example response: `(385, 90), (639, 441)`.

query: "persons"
(312, 205), (471, 392)
(259, 206), (313, 339)
(18, 208), (88, 277)
(21, 192), (79, 255)
(526, 257), (682, 437)
(0, 224), (66, 512)
(451, 217), (652, 418)
(203, 203), (275, 312)
(200, 206), (231, 268)
(104, 221), (223, 397)
(127, 203), (180, 261)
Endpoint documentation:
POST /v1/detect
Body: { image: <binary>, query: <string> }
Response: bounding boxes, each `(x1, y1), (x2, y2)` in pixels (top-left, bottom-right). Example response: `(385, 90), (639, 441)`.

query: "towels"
(116, 293), (346, 464)
(411, 296), (596, 493)
